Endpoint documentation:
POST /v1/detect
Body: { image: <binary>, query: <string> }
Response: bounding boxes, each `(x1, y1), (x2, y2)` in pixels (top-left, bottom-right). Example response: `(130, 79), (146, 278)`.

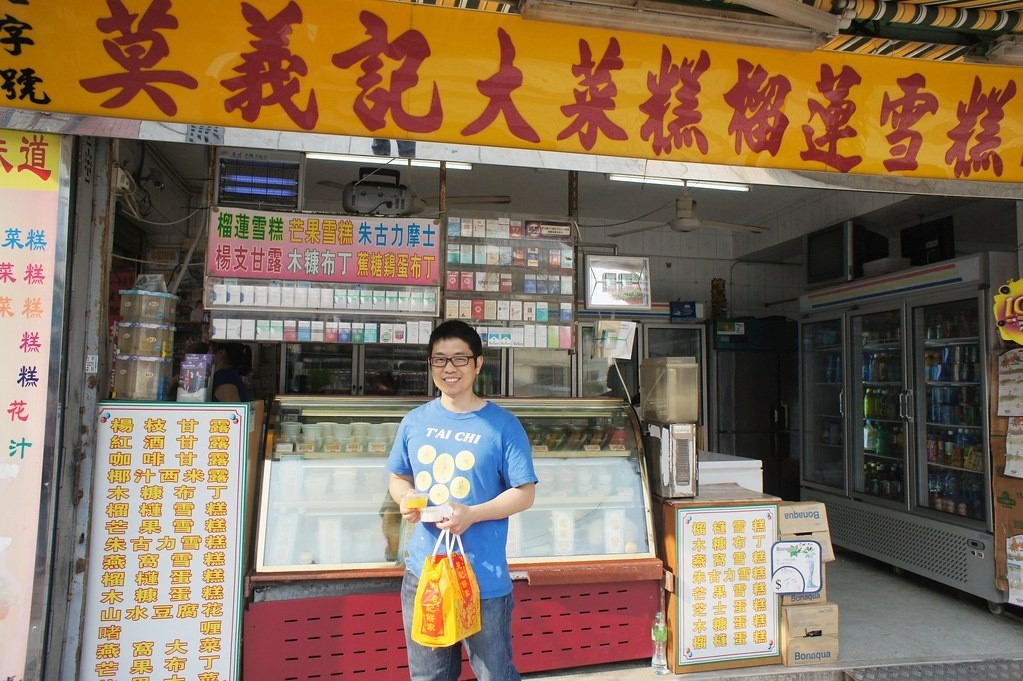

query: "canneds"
(474, 364), (496, 397)
(923, 320), (980, 426)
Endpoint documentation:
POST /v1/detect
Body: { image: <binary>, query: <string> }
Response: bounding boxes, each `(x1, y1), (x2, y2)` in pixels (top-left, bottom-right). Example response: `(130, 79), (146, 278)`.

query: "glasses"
(429, 355), (478, 367)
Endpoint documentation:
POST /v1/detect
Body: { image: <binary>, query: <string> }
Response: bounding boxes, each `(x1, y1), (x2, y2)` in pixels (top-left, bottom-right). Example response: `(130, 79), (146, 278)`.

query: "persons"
(209, 342), (252, 402)
(386, 320), (539, 681)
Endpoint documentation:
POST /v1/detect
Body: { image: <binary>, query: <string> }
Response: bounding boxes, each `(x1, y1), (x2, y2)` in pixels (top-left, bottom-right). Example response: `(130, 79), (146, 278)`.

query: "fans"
(317, 159), (513, 214)
(603, 180), (770, 237)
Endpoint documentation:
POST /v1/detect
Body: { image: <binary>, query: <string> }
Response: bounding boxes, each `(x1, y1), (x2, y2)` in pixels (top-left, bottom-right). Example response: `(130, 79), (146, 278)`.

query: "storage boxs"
(639, 356), (700, 425)
(212, 218), (573, 349)
(862, 257), (911, 278)
(777, 500), (841, 666)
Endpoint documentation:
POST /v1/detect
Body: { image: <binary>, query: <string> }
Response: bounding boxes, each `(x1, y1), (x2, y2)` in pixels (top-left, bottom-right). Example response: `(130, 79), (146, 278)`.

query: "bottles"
(862, 310), (985, 521)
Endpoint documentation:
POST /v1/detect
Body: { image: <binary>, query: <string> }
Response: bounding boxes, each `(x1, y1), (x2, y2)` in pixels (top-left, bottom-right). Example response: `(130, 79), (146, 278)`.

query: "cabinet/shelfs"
(202, 236), (576, 351)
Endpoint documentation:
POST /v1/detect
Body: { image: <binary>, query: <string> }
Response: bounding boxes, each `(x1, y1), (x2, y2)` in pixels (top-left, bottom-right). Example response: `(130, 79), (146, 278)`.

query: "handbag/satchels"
(411, 528), (482, 648)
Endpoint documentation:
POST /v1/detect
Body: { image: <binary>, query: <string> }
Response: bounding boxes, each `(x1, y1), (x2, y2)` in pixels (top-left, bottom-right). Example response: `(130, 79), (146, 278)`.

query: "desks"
(653, 481), (780, 675)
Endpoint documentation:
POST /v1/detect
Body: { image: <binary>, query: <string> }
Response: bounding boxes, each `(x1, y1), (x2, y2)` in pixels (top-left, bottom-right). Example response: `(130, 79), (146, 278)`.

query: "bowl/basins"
(404, 493), (429, 509)
(420, 506), (443, 522)
(280, 421), (400, 453)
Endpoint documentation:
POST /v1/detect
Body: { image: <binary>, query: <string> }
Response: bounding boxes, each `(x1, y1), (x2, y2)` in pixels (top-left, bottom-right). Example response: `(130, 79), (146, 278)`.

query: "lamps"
(213, 147), (307, 213)
(517, 0), (839, 51)
(985, 34), (1023, 65)
